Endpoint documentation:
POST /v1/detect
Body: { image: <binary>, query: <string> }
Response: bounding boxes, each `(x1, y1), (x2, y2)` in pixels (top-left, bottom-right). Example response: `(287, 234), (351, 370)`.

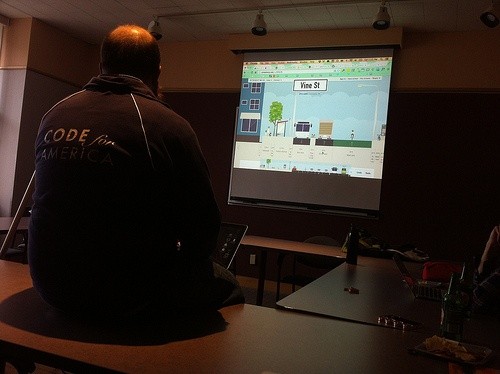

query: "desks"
(0, 218), (500, 374)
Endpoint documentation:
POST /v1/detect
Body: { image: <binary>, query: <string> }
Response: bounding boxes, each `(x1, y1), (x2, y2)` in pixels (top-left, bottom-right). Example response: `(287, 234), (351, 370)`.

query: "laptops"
(211, 221), (247, 268)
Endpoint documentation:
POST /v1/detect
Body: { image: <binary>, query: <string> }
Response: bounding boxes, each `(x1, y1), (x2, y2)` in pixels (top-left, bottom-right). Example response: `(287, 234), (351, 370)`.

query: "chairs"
(276, 235), (341, 301)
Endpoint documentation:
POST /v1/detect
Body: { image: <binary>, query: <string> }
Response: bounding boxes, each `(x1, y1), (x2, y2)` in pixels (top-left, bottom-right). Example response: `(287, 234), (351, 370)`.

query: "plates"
(414, 335), (492, 365)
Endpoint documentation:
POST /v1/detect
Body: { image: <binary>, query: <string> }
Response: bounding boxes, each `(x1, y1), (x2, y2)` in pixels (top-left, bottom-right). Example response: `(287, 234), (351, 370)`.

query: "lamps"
(250, 13), (267, 36)
(479, 1), (500, 28)
(148, 20), (163, 40)
(372, 6), (390, 30)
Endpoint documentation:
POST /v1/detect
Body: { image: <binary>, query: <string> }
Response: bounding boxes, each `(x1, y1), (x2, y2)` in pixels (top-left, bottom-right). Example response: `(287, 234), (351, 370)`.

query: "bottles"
(439, 272), (461, 338)
(345, 223), (359, 264)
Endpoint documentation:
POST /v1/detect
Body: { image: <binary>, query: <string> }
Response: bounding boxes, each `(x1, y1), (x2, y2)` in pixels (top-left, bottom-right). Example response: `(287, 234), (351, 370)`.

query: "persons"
(26, 24), (245, 331)
